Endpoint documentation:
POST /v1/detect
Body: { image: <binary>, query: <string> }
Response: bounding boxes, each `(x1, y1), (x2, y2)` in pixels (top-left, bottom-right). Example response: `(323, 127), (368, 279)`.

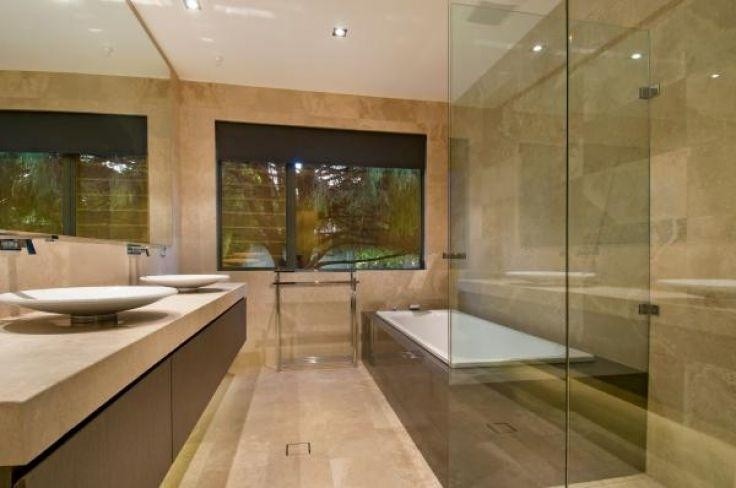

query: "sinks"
(654, 278), (736, 299)
(139, 273), (231, 293)
(503, 271), (598, 285)
(0, 284), (178, 325)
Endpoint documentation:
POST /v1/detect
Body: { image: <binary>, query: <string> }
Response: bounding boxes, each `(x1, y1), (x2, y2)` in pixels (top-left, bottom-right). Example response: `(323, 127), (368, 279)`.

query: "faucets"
(152, 243), (167, 257)
(0, 236), (37, 256)
(126, 243), (150, 257)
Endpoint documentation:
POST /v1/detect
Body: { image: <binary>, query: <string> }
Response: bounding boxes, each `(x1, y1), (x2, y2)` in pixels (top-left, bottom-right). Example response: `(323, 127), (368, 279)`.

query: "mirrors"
(1, 0), (186, 249)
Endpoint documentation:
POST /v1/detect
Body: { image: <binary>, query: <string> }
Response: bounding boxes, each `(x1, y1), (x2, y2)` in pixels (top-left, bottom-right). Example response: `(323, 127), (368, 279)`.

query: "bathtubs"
(374, 307), (595, 369)
